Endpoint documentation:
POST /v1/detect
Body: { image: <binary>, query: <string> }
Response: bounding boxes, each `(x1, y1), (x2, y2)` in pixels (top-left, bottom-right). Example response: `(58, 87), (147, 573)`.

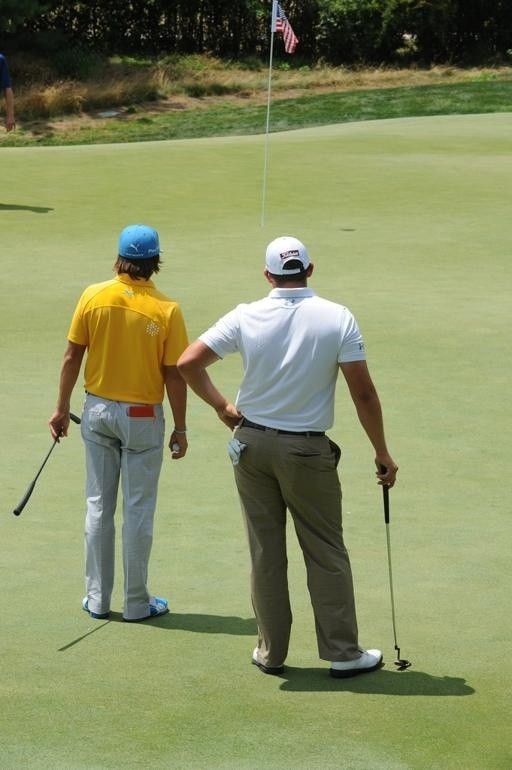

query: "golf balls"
(172, 444), (181, 453)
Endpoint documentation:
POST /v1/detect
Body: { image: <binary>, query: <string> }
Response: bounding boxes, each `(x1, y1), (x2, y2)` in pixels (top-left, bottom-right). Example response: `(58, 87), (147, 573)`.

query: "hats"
(116, 223), (165, 260)
(264, 235), (310, 277)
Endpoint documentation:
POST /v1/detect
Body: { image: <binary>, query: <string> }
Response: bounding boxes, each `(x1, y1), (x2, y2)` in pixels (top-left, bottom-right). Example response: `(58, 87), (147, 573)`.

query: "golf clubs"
(379, 466), (411, 667)
(14, 414), (82, 516)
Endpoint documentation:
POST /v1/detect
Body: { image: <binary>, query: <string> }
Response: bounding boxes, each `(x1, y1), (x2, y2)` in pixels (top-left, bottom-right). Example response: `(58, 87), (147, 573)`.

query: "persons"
(46, 222), (190, 627)
(0, 54), (16, 133)
(174, 234), (399, 679)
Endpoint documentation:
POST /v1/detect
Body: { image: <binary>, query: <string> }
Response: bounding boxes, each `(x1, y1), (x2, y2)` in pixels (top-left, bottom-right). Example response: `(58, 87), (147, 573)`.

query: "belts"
(241, 419), (326, 438)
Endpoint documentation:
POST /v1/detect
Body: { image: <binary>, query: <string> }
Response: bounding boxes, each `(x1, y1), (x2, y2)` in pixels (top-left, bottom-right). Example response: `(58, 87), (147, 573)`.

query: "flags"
(271, 0), (300, 56)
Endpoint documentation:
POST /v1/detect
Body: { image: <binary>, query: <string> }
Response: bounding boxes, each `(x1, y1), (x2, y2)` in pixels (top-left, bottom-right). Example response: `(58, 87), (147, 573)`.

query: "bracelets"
(173, 424), (189, 434)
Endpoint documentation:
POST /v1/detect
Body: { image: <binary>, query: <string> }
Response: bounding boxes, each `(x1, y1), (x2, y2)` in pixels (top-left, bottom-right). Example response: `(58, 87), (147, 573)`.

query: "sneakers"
(251, 646), (285, 675)
(122, 596), (169, 623)
(329, 648), (384, 680)
(82, 596), (109, 620)
